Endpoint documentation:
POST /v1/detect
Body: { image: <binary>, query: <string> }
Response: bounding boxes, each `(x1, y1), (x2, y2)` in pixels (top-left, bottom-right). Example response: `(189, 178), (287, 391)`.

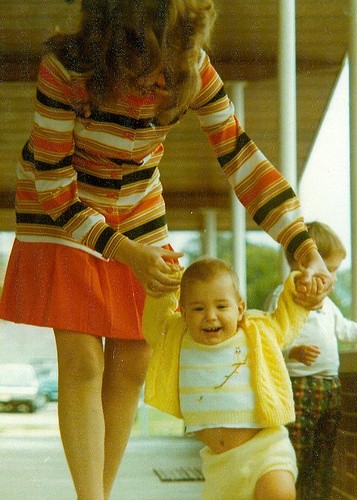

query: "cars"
(1, 363), (58, 413)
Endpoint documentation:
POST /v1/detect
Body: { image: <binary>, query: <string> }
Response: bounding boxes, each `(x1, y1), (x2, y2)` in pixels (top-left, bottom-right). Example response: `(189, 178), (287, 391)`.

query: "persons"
(1, 1), (337, 500)
(265, 221), (357, 500)
(140, 261), (334, 500)
